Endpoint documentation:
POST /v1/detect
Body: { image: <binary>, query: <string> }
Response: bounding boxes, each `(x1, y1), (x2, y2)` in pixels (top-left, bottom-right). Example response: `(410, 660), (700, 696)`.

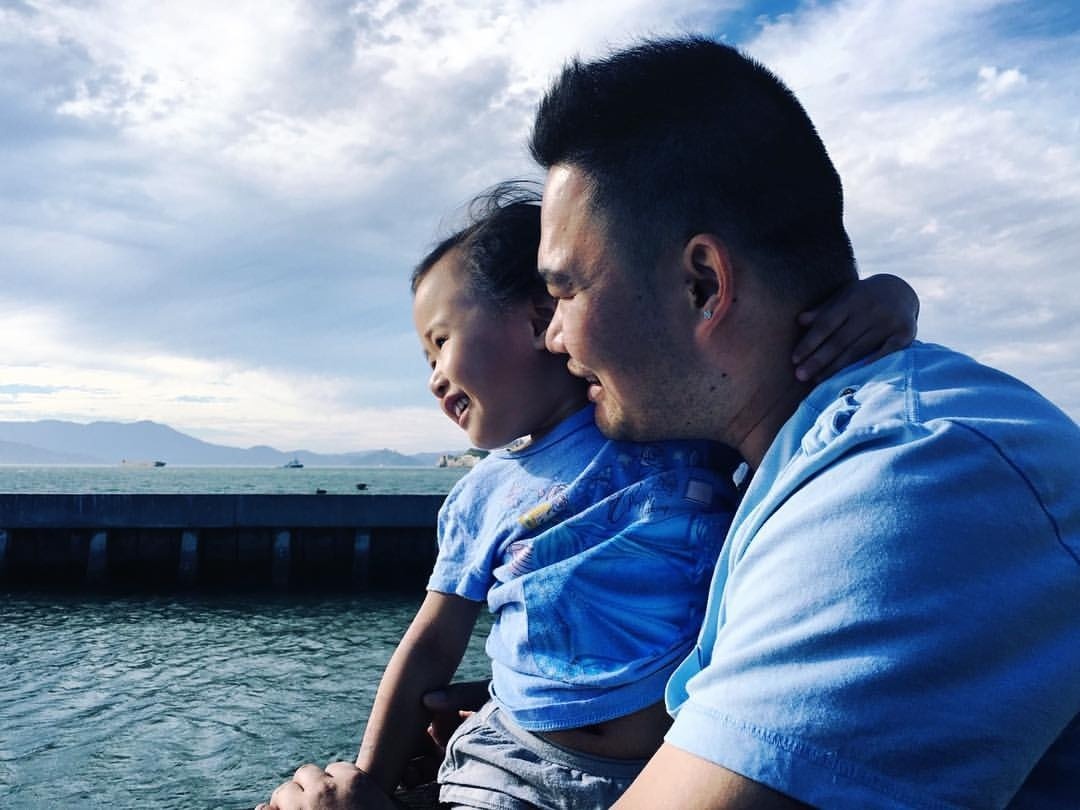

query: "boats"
(284, 459), (304, 468)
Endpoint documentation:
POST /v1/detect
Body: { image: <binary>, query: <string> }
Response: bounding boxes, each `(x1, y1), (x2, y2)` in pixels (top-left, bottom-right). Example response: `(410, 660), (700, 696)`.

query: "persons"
(256, 38), (1080, 810)
